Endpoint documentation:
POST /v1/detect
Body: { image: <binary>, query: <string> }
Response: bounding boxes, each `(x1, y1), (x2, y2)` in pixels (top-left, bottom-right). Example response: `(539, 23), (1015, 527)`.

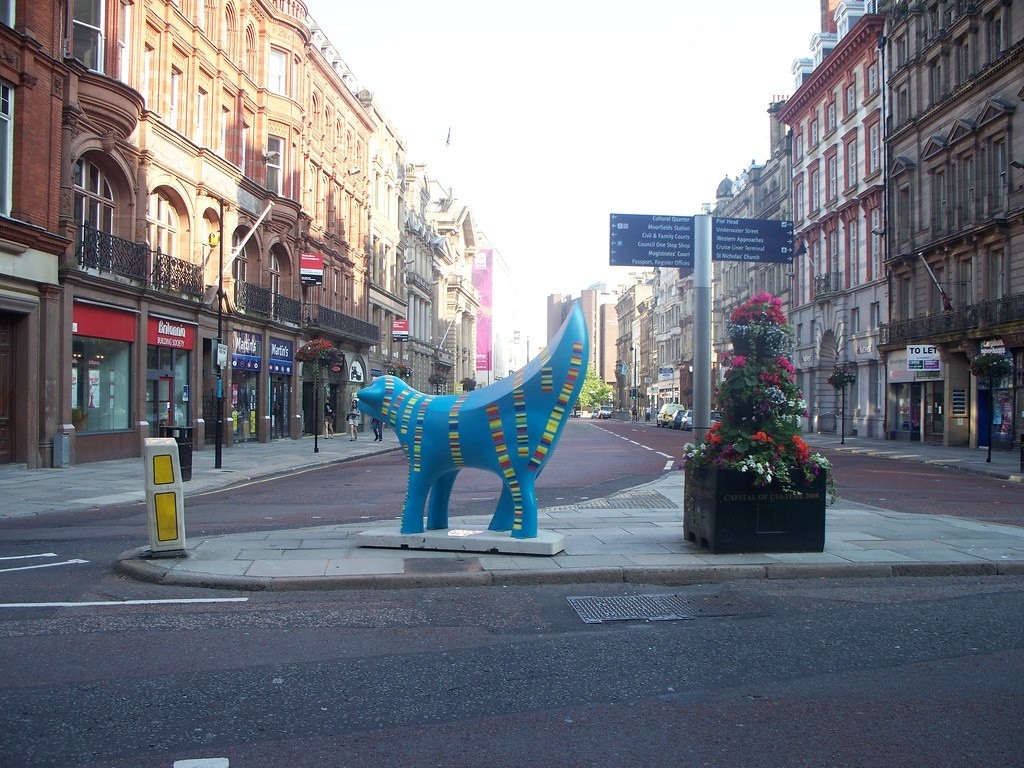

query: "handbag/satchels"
(634, 411), (636, 415)
(346, 415), (349, 421)
(331, 413), (335, 418)
(352, 415), (356, 418)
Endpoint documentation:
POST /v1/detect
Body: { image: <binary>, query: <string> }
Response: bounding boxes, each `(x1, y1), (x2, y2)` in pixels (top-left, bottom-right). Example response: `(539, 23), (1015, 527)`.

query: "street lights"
(629, 341), (637, 423)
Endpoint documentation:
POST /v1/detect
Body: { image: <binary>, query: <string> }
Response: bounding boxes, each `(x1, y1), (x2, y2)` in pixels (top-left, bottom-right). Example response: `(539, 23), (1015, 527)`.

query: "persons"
(324, 397), (336, 439)
(347, 400), (361, 441)
(370, 418), (383, 441)
(645, 405), (651, 421)
(630, 406), (637, 423)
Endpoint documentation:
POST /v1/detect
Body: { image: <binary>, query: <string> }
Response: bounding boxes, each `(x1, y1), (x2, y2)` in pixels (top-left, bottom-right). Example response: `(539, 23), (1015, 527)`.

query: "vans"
(656, 404), (684, 428)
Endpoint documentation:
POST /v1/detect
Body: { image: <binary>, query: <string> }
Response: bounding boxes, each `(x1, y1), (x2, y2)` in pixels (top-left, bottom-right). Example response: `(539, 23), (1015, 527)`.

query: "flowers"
(678, 290), (837, 505)
(969, 353), (1012, 387)
(295, 338), (338, 392)
(387, 363), (406, 379)
(827, 365), (856, 390)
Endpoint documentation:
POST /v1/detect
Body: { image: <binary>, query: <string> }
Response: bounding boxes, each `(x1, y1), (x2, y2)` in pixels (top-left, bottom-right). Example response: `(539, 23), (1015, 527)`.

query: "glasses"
(647, 406), (649, 407)
(353, 402), (357, 403)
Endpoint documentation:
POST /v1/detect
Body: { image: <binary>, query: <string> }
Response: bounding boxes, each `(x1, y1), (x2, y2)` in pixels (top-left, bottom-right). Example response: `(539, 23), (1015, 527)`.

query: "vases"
(683, 466), (825, 554)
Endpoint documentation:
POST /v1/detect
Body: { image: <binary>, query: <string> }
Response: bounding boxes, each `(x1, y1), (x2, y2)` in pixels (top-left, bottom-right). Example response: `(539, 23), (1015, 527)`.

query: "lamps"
(870, 226), (882, 235)
(266, 151), (281, 160)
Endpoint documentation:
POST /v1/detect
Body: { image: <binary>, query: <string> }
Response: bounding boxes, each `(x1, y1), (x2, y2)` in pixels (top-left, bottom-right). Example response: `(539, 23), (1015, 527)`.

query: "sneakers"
(632, 421), (636, 422)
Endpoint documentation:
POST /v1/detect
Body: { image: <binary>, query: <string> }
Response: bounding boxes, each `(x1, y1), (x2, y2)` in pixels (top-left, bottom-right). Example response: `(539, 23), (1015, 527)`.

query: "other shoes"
(325, 435), (333, 438)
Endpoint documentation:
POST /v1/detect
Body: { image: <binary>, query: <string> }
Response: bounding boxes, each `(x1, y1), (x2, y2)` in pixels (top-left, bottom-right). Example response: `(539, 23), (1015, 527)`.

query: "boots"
(350, 436), (357, 441)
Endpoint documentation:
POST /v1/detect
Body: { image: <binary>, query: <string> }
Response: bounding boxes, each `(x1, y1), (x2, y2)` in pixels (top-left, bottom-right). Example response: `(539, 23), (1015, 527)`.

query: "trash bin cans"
(158, 426), (197, 482)
(52, 432), (71, 470)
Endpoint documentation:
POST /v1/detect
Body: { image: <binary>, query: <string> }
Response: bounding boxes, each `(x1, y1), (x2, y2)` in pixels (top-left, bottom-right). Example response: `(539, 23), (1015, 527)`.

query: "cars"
(591, 406), (613, 419)
(569, 412), (580, 418)
(680, 409), (693, 431)
(710, 410), (725, 428)
(667, 409), (686, 429)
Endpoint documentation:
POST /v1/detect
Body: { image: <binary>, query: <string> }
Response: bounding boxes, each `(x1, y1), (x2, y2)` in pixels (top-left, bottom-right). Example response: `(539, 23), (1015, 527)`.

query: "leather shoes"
(375, 437), (382, 441)
